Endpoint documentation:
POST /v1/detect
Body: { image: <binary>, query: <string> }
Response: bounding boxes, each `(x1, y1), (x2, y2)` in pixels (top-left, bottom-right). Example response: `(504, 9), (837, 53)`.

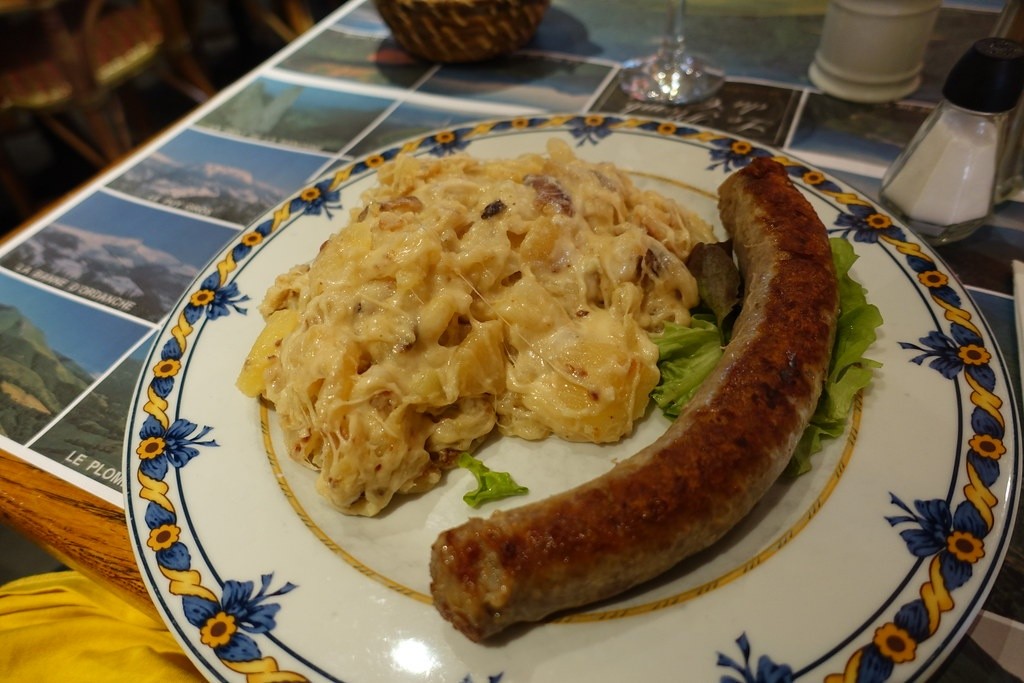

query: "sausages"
(426, 156), (841, 641)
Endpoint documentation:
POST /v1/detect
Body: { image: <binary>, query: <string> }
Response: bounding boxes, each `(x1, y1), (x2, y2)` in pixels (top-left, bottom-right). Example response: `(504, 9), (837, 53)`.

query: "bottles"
(879, 37), (1024, 246)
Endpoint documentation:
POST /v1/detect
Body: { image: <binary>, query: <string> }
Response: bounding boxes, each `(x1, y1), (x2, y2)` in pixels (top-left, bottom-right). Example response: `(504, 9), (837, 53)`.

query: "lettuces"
(456, 453), (531, 508)
(644, 230), (882, 477)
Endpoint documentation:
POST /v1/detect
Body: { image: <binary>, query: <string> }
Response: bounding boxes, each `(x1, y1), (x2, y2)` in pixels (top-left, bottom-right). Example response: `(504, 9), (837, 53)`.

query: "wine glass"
(620, 0), (727, 105)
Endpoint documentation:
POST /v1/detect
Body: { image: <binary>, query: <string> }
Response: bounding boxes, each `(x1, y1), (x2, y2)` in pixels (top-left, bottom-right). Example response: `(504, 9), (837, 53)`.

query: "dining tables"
(0, 0), (1024, 683)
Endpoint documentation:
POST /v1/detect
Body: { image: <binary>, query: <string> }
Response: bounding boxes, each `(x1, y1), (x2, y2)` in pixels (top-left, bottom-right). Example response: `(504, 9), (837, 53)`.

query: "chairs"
(0, 1), (328, 226)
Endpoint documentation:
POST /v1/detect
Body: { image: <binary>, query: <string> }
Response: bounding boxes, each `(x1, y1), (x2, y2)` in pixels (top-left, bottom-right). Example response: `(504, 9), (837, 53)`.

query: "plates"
(122, 113), (1024, 683)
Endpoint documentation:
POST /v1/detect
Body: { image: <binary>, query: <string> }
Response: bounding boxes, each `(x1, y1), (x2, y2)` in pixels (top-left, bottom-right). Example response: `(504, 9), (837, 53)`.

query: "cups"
(809, 0), (942, 103)
